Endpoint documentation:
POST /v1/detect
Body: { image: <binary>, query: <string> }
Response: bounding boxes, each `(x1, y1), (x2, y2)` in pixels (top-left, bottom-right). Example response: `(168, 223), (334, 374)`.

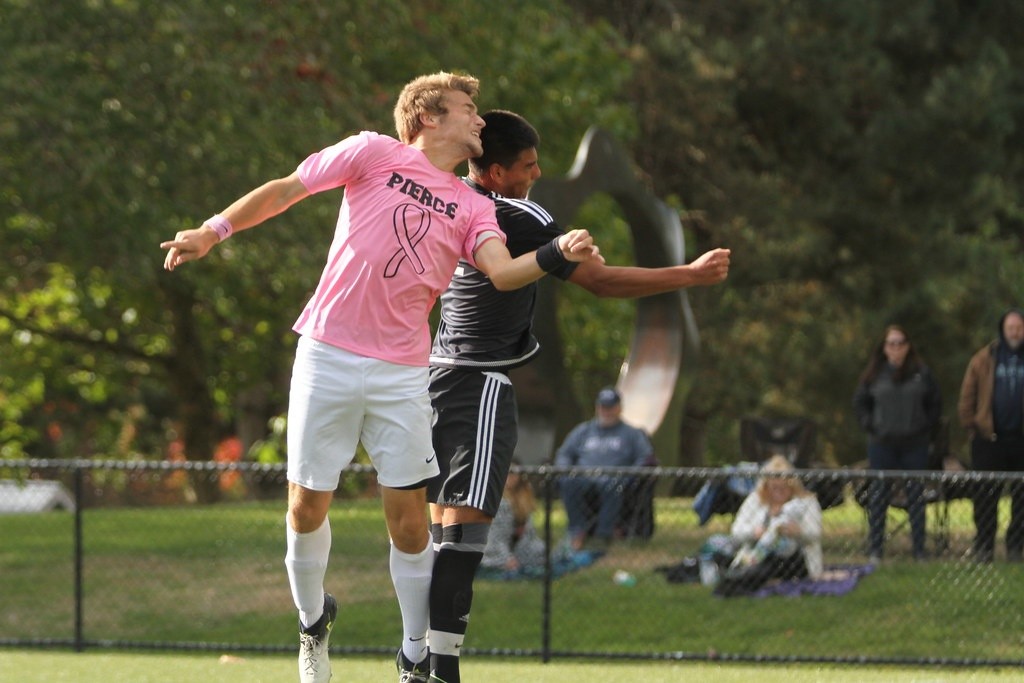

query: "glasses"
(885, 340), (908, 347)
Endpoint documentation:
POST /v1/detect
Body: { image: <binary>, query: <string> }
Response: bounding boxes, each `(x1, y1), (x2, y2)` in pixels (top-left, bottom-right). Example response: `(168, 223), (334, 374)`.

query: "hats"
(597, 388), (621, 407)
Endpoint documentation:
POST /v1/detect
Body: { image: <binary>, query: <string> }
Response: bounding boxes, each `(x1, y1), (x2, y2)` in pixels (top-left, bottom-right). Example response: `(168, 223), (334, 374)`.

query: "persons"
(552, 389), (656, 551)
(731, 497), (804, 565)
(155, 71), (592, 683)
(957, 309), (1024, 562)
(701, 453), (823, 599)
(480, 455), (564, 573)
(430, 106), (731, 674)
(858, 327), (941, 562)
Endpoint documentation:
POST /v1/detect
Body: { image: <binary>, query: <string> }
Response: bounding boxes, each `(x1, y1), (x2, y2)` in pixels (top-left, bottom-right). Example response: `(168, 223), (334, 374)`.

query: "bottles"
(696, 528), (797, 584)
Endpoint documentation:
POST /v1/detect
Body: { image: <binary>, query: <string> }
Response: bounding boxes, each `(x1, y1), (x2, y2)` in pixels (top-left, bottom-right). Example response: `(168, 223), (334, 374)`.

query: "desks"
(689, 463), (845, 531)
(851, 457), (971, 554)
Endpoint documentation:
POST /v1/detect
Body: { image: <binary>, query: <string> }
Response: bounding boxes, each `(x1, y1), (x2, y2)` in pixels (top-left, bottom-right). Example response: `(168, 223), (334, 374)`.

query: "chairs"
(581, 462), (663, 546)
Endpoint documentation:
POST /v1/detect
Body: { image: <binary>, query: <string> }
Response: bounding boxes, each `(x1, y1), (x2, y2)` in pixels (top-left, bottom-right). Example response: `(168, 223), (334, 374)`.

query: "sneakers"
(395, 646), (430, 683)
(298, 593), (339, 683)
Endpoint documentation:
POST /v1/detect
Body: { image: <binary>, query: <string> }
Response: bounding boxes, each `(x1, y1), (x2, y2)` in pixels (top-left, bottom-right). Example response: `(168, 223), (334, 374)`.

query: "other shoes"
(869, 554), (883, 566)
(1005, 554), (1023, 565)
(976, 550), (995, 566)
(430, 672), (448, 683)
